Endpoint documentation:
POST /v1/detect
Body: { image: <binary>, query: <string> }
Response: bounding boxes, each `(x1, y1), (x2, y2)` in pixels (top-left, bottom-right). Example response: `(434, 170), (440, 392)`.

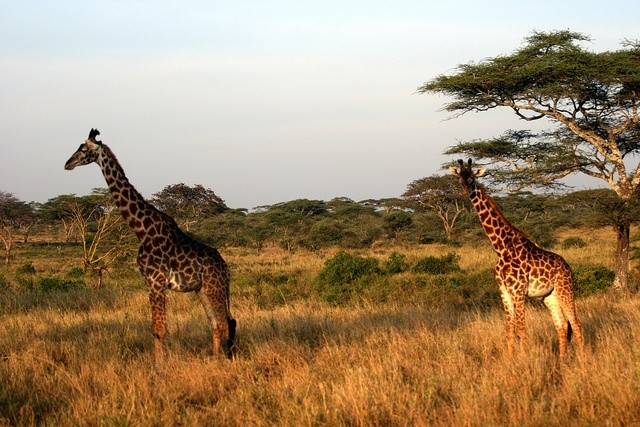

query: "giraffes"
(64, 128), (236, 360)
(449, 157), (585, 364)
